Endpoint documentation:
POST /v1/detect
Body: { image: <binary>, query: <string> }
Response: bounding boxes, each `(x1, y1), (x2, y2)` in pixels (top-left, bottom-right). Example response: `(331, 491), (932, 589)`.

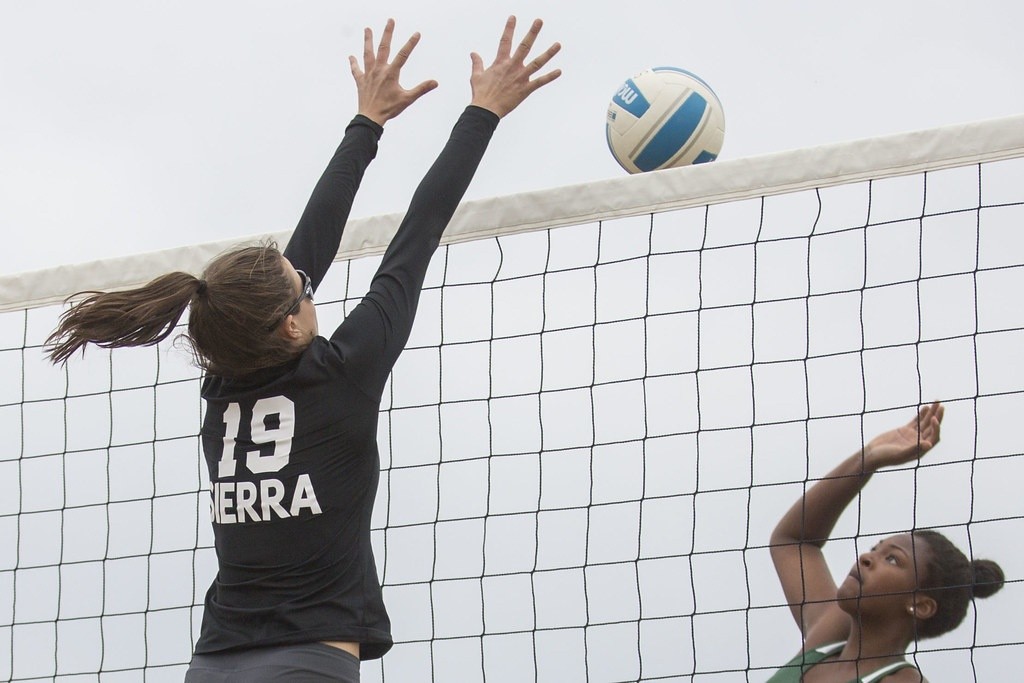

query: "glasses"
(265, 269), (314, 334)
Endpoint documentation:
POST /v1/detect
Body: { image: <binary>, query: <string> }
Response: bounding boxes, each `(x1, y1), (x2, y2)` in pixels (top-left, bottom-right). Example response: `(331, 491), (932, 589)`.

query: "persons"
(762, 397), (1008, 683)
(38, 12), (565, 683)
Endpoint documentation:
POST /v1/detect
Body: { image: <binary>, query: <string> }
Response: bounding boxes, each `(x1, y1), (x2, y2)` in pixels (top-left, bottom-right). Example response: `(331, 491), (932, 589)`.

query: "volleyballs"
(604, 65), (727, 175)
